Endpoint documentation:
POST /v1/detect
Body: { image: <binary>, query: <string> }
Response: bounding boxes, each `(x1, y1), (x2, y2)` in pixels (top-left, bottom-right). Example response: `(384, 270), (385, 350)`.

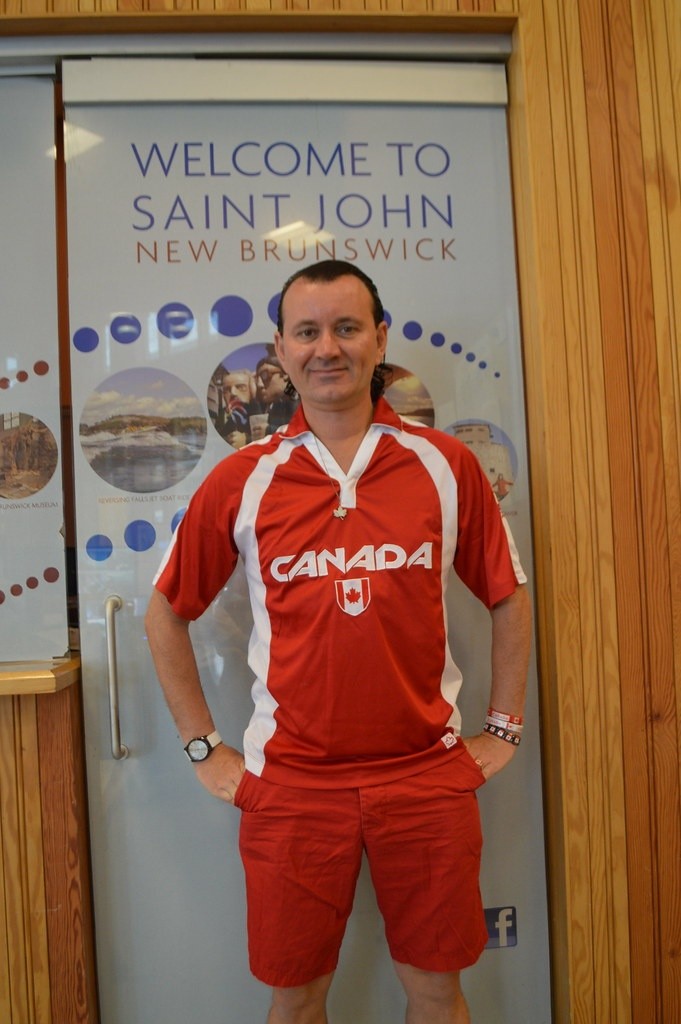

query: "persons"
(214, 357), (301, 449)
(144, 260), (534, 1024)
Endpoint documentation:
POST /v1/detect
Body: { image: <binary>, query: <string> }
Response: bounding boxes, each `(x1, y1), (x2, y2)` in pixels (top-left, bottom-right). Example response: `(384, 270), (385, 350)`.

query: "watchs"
(184, 731), (223, 761)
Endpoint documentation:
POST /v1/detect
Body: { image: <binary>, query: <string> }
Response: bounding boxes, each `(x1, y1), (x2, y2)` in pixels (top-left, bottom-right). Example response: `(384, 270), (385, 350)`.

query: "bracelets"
(483, 707), (523, 747)
